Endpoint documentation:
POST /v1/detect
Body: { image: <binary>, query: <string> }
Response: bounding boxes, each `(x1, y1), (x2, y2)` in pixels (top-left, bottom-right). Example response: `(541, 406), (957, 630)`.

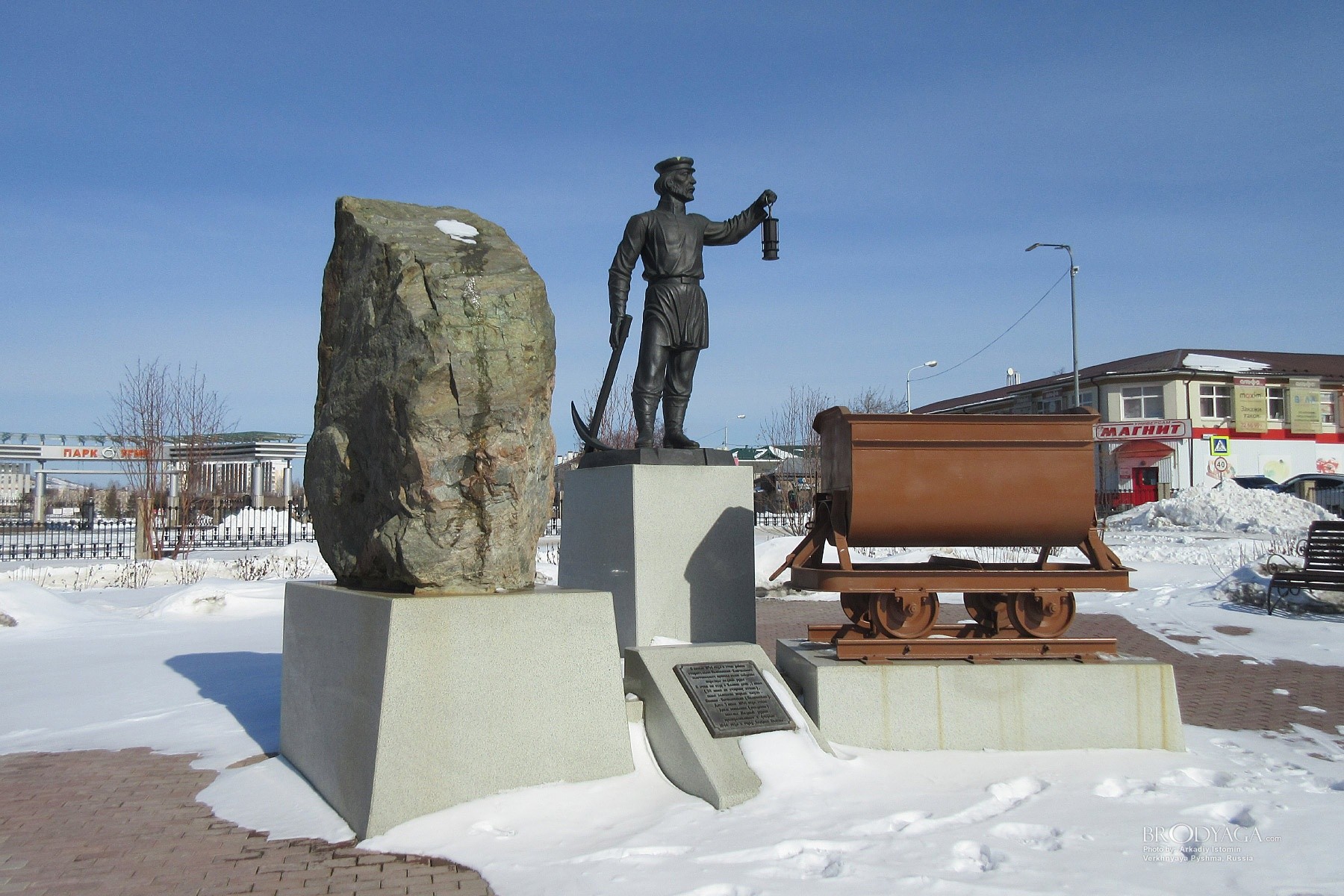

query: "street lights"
(1025, 242), (1081, 408)
(724, 415), (746, 446)
(907, 360), (938, 415)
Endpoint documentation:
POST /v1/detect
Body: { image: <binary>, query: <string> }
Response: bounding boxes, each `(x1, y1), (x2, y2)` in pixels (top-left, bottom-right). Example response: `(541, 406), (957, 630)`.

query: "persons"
(607, 157), (777, 449)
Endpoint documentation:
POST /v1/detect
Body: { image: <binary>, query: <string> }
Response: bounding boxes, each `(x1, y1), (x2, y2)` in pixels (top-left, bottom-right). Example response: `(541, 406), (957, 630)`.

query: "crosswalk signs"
(1213, 439), (1227, 453)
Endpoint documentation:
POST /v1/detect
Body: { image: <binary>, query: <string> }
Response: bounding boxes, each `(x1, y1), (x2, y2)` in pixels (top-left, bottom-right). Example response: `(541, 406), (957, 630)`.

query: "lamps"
(1215, 416), (1231, 428)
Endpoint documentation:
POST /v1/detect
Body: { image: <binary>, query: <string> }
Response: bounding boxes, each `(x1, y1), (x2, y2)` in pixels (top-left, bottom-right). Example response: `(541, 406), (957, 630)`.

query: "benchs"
(1266, 521), (1344, 615)
